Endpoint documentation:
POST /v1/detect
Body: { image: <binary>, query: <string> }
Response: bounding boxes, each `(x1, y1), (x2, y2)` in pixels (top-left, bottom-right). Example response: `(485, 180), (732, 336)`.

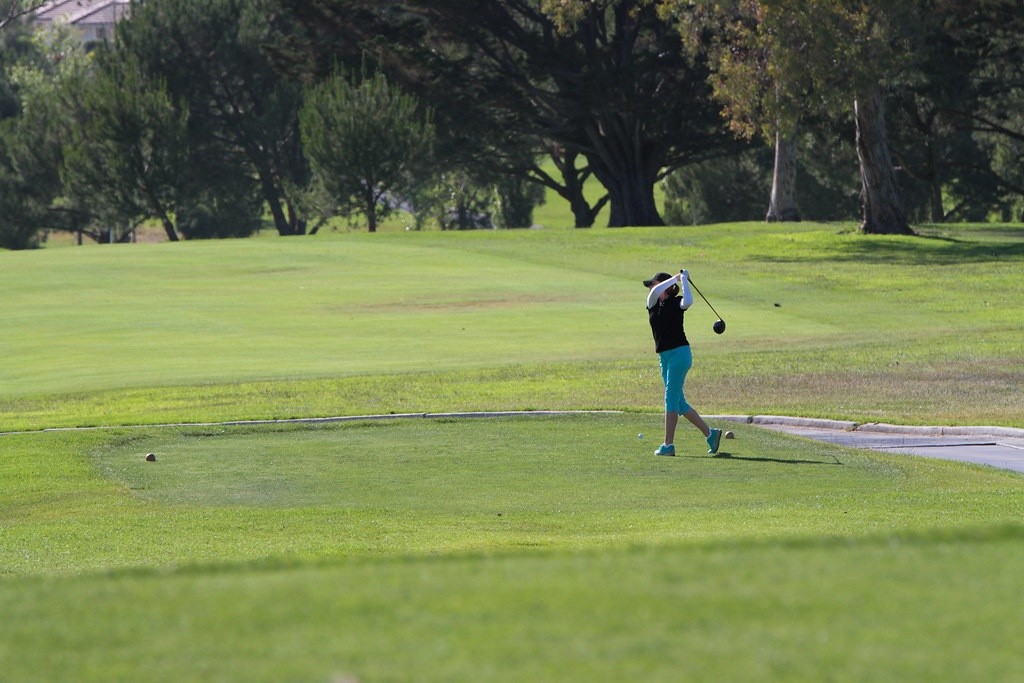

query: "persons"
(643, 272), (723, 456)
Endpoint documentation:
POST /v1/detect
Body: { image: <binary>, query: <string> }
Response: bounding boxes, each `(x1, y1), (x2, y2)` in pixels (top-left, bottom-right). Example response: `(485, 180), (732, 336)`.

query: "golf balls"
(638, 433), (643, 438)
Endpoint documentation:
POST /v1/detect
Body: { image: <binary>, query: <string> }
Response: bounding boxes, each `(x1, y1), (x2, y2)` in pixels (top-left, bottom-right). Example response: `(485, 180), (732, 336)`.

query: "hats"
(643, 272), (674, 287)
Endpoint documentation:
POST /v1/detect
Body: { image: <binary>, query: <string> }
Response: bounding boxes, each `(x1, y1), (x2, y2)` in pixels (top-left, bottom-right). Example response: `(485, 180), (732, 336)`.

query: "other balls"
(146, 453), (155, 461)
(725, 431), (734, 439)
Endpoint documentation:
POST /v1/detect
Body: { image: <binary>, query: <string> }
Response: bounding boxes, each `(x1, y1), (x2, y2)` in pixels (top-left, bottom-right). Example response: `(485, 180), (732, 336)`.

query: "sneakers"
(654, 444), (675, 456)
(706, 429), (722, 453)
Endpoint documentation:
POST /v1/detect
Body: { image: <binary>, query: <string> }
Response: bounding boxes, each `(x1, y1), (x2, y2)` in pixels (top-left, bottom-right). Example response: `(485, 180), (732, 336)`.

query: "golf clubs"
(680, 269), (725, 334)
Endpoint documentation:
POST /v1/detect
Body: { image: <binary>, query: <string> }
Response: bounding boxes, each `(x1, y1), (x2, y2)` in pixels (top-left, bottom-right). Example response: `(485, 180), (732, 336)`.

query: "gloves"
(680, 269), (689, 278)
